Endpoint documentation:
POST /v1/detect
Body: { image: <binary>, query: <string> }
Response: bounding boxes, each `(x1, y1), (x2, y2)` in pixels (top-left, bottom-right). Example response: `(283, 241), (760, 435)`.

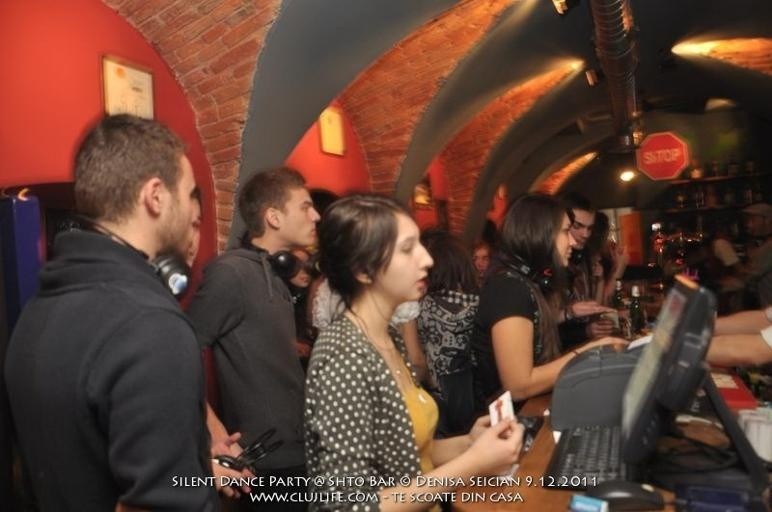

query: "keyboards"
(542, 425), (632, 490)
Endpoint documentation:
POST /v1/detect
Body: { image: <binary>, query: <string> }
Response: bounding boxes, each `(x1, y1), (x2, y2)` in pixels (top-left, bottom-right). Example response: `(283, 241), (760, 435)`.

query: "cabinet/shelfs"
(664, 170), (772, 261)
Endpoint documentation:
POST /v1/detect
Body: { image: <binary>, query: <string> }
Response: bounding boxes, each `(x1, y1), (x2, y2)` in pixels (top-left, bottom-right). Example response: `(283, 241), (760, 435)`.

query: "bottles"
(613, 278), (624, 309)
(629, 280), (645, 336)
(688, 157), (760, 181)
(675, 186), (754, 210)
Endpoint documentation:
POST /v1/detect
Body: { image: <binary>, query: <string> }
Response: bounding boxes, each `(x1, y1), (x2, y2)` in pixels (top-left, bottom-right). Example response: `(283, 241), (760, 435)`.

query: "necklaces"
(362, 327), (408, 382)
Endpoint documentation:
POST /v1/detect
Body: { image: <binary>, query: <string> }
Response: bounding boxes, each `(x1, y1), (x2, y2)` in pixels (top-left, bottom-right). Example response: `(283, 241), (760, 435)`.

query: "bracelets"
(572, 347), (578, 355)
(561, 305), (571, 322)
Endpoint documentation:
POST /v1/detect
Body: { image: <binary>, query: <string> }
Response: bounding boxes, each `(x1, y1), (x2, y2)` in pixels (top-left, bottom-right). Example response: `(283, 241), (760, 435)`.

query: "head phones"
(251, 244), (300, 278)
(69, 220), (191, 300)
(507, 252), (552, 290)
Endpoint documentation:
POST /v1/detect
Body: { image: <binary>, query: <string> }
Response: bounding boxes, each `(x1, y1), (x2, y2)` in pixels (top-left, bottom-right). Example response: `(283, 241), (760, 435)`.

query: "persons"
(4, 112), (223, 510)
(150, 182), (258, 500)
(301, 199), (529, 512)
(279, 192), (771, 441)
(184, 167), (323, 510)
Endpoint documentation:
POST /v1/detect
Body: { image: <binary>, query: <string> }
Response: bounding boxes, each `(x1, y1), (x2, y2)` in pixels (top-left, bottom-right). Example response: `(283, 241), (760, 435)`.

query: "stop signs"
(636, 133), (690, 180)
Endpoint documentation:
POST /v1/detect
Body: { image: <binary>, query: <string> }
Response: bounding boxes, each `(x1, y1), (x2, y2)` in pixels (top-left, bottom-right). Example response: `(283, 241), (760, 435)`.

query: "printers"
(551, 343), (644, 431)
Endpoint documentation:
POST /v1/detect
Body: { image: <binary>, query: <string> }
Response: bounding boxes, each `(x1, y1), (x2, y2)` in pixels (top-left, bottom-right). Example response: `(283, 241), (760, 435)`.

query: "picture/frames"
(412, 170), (437, 213)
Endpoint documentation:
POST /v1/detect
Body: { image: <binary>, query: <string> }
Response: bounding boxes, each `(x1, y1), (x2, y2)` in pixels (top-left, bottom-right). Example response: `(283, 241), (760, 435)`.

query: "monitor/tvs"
(619, 274), (716, 467)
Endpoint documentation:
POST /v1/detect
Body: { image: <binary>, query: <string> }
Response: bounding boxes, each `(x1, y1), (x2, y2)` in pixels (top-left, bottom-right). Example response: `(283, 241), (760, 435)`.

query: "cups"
(736, 404), (771, 462)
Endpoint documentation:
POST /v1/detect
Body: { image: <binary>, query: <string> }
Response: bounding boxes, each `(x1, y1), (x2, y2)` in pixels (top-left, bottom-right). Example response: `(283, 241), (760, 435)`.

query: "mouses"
(585, 480), (663, 511)
